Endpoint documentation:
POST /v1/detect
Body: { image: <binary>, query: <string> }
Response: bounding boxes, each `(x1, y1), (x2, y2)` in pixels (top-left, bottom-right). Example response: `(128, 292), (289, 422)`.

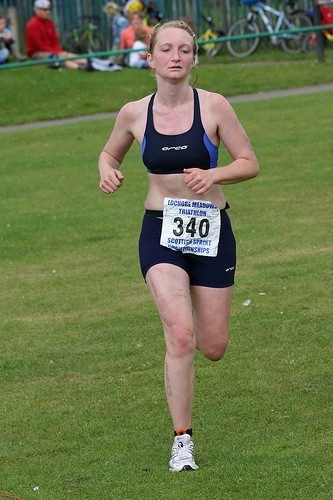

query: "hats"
(124, 0), (143, 17)
(34, 0), (50, 9)
(102, 2), (119, 11)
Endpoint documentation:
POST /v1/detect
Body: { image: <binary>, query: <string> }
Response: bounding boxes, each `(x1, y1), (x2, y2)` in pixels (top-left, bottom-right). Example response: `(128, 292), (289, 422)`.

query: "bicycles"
(225, 0), (314, 58)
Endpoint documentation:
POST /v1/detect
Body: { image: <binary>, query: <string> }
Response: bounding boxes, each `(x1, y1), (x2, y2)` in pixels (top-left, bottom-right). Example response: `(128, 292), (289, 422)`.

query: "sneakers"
(168, 434), (198, 472)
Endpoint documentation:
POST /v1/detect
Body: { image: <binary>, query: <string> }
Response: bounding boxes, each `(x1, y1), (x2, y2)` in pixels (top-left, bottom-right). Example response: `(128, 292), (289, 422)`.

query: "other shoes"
(86, 57), (94, 71)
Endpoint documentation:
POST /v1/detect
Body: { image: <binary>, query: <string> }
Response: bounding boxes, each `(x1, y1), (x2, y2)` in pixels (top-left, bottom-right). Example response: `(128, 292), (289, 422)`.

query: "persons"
(98, 20), (258, 473)
(0, 0), (159, 71)
